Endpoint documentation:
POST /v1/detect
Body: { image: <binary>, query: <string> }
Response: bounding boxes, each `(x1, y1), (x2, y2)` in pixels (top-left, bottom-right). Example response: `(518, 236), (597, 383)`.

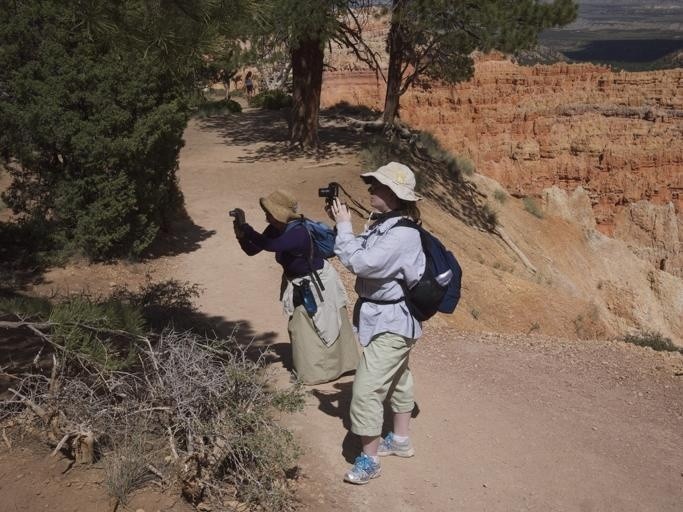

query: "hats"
(362, 162), (423, 202)
(260, 193), (303, 225)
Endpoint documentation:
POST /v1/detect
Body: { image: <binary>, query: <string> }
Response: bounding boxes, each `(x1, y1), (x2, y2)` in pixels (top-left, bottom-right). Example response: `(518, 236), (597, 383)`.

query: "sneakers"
(377, 431), (414, 457)
(345, 451), (382, 485)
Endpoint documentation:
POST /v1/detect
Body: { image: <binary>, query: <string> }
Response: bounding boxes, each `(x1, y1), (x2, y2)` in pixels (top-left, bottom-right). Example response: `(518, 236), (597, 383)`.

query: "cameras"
(318, 183), (338, 206)
(229, 210), (240, 216)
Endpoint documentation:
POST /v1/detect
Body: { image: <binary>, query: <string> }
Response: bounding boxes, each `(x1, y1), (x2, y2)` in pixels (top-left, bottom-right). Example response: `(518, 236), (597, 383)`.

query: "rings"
(334, 210), (339, 214)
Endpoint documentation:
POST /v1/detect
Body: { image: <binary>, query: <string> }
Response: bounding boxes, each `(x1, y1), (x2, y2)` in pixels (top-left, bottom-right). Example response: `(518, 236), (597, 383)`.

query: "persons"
(232, 75), (242, 90)
(328, 161), (427, 483)
(222, 79), (231, 101)
(243, 71), (252, 97)
(228, 190), (362, 384)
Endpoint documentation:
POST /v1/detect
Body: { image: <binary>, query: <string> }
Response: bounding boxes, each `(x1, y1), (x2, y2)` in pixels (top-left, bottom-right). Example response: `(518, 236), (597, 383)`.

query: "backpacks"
(391, 219), (461, 321)
(300, 215), (336, 260)
